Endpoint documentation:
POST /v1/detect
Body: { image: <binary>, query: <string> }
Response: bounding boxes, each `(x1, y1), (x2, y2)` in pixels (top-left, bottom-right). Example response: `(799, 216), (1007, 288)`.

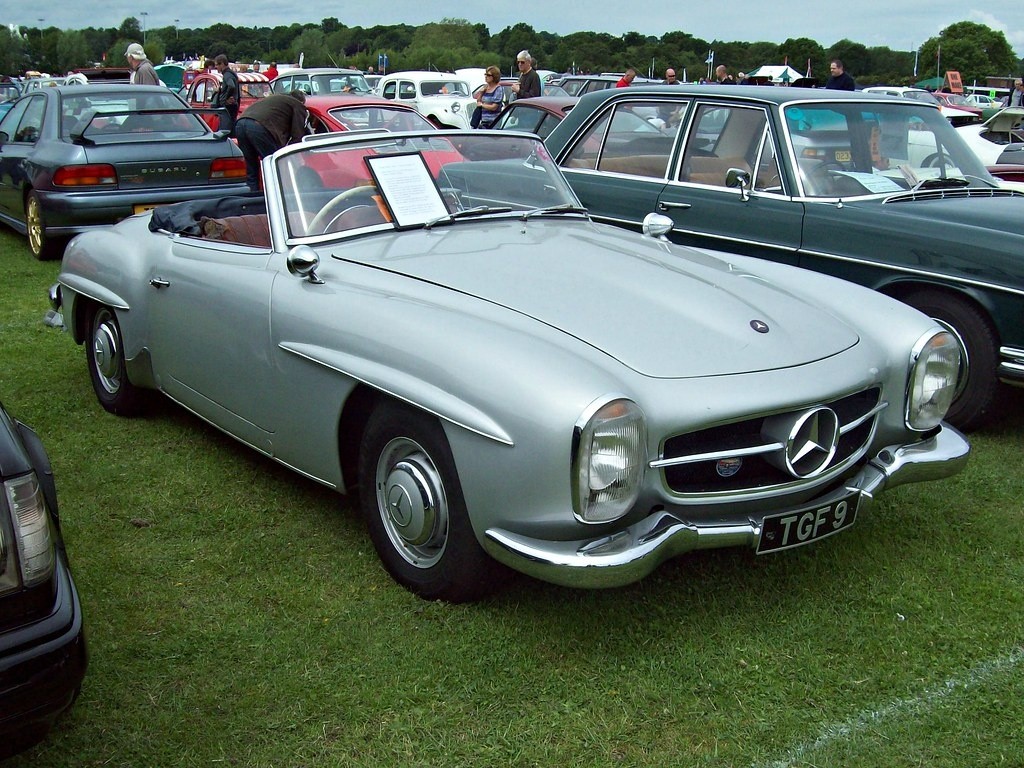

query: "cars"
(0, 68), (1024, 434)
(0, 399), (87, 760)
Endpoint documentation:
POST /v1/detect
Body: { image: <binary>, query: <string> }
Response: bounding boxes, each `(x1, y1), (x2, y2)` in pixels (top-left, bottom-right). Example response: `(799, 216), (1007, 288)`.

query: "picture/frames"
(363, 150), (456, 232)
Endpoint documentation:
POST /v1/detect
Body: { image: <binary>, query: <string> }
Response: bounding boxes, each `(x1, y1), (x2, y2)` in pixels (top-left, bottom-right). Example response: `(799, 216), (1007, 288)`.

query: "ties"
(28, 135), (31, 140)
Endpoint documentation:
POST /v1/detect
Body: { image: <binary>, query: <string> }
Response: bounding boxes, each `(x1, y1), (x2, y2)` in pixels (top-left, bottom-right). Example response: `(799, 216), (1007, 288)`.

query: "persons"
(378, 65), (384, 75)
(473, 66), (504, 130)
(202, 60), (215, 74)
(367, 67), (376, 74)
(253, 61), (260, 74)
(656, 68), (681, 122)
(235, 89), (307, 191)
(1018, 83), (1024, 108)
(616, 69), (635, 111)
(263, 62), (278, 81)
(240, 65), (247, 73)
(1008, 78), (1022, 107)
(214, 55), (240, 139)
(698, 65), (775, 86)
(827, 60), (856, 92)
(511, 50), (541, 127)
(126, 43), (163, 108)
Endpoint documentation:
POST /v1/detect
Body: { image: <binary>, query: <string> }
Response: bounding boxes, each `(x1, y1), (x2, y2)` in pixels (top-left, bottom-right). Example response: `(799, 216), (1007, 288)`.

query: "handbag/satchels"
(470, 85), (488, 128)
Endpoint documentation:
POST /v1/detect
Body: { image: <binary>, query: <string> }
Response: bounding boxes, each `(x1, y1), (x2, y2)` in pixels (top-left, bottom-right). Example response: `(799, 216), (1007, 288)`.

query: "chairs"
(336, 207), (383, 231)
(765, 158), (836, 197)
(62, 114), (77, 136)
(121, 116), (156, 131)
(690, 171), (726, 186)
(205, 210), (329, 245)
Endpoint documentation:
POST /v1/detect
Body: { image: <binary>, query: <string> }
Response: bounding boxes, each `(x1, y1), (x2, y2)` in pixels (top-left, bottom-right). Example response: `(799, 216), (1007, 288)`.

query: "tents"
(745, 65), (803, 83)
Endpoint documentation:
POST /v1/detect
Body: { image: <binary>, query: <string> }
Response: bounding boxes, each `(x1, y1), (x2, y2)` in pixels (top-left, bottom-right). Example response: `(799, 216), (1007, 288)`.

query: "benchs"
(587, 154), (752, 181)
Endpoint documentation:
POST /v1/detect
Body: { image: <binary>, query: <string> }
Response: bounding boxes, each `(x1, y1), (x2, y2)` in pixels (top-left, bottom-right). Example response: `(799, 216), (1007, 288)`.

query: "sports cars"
(45, 128), (971, 602)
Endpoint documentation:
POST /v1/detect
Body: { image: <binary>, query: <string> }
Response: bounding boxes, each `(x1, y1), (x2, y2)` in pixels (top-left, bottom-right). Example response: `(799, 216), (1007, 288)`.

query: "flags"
(705, 53), (713, 63)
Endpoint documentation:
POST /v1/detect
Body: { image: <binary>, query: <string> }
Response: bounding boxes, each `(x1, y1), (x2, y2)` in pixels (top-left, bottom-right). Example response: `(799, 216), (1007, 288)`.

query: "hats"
(127, 43), (146, 60)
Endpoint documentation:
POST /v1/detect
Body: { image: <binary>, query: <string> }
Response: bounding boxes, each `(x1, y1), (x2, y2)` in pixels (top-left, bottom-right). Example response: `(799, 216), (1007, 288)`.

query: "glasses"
(669, 75), (675, 77)
(26, 130), (34, 131)
(485, 74), (493, 76)
(517, 61), (525, 64)
(215, 64), (219, 66)
